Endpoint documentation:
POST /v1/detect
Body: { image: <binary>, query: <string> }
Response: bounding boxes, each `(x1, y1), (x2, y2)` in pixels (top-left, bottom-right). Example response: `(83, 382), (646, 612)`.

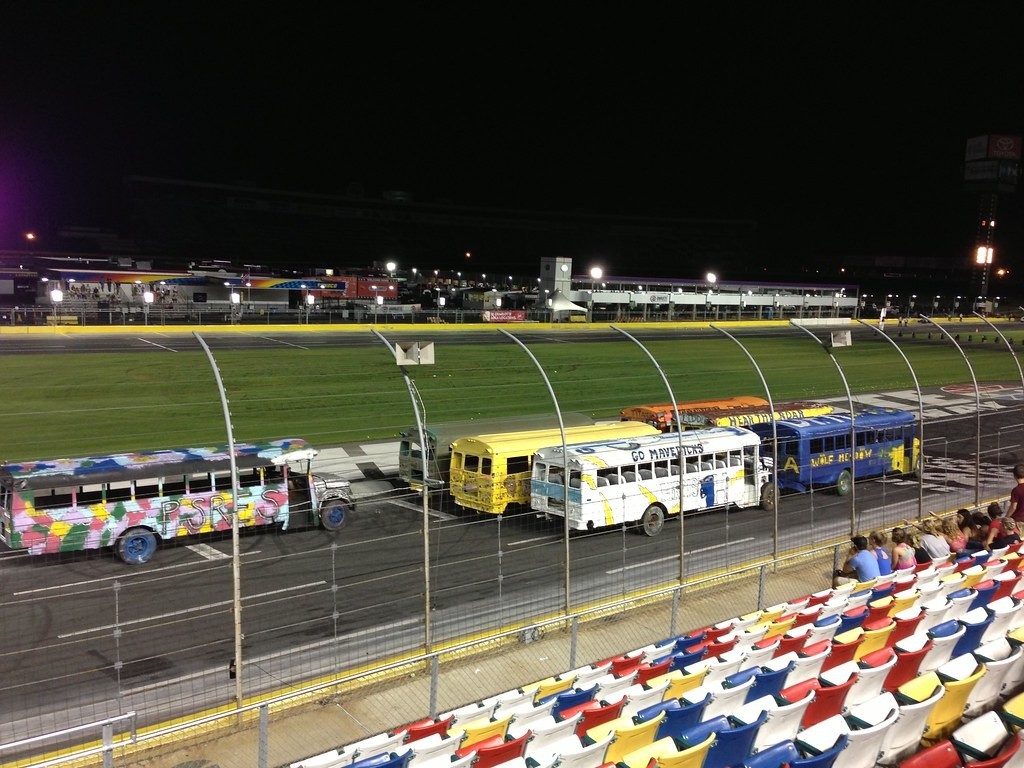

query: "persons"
(960, 313), (963, 321)
(64, 275), (120, 299)
(948, 314), (952, 321)
(833, 463), (1024, 588)
(898, 315), (908, 327)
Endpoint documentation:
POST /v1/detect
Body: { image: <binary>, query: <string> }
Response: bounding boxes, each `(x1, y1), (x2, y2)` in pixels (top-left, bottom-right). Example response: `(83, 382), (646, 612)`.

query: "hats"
(851, 535), (867, 549)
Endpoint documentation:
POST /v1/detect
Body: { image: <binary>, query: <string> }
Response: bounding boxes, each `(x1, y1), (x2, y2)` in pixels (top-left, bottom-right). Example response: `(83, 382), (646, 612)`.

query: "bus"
(397, 411), (781, 537)
(0, 438), (358, 566)
(618, 395), (926, 502)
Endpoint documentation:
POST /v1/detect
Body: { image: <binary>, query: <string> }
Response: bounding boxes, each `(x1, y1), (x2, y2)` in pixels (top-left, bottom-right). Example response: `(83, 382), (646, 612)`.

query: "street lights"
(300, 284), (325, 324)
(41, 277), (76, 326)
(134, 280), (166, 325)
(371, 284), (395, 323)
(543, 289), (550, 322)
(435, 287), (457, 324)
(223, 281), (251, 325)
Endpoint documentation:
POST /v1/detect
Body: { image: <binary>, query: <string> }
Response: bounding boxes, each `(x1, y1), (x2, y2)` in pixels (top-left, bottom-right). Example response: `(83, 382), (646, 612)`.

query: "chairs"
(542, 457), (741, 488)
(335, 541), (1024, 768)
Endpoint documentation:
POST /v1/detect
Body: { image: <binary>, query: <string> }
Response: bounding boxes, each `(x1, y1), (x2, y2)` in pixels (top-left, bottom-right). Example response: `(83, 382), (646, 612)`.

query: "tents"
(532, 290), (587, 323)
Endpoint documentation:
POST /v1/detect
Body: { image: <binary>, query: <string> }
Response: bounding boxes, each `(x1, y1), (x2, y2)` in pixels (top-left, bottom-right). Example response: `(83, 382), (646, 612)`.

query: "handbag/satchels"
(834, 569), (847, 577)
